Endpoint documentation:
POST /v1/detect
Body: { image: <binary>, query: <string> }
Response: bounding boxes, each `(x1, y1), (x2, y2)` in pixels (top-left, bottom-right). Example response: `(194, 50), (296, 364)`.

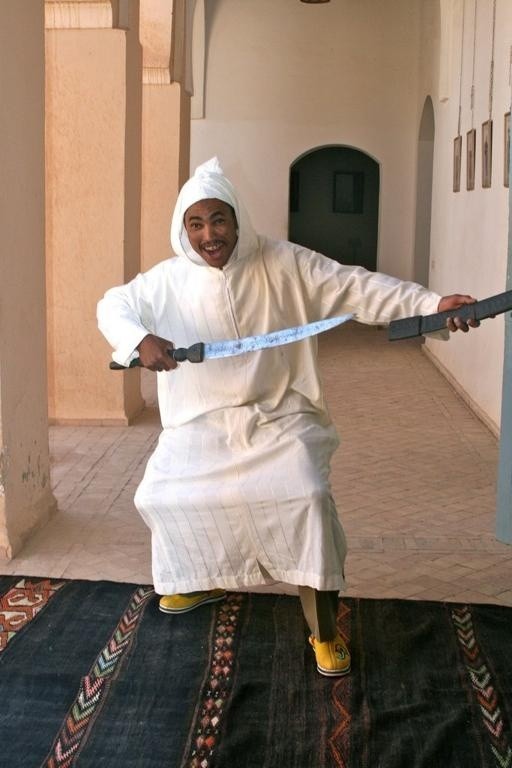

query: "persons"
(96, 155), (495, 676)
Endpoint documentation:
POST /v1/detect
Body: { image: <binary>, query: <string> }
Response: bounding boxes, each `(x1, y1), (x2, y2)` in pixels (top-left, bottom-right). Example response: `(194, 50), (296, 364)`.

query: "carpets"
(0, 574), (512, 768)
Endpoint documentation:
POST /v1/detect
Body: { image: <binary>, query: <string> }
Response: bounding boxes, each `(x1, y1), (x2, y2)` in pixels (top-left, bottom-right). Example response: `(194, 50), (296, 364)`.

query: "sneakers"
(159, 588), (227, 614)
(309, 633), (351, 677)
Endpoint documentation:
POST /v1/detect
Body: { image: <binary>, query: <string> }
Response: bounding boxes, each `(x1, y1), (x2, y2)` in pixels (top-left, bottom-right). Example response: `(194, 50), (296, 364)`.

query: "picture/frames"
(467, 129), (475, 190)
(453, 136), (462, 192)
(504, 112), (511, 188)
(482, 120), (491, 188)
